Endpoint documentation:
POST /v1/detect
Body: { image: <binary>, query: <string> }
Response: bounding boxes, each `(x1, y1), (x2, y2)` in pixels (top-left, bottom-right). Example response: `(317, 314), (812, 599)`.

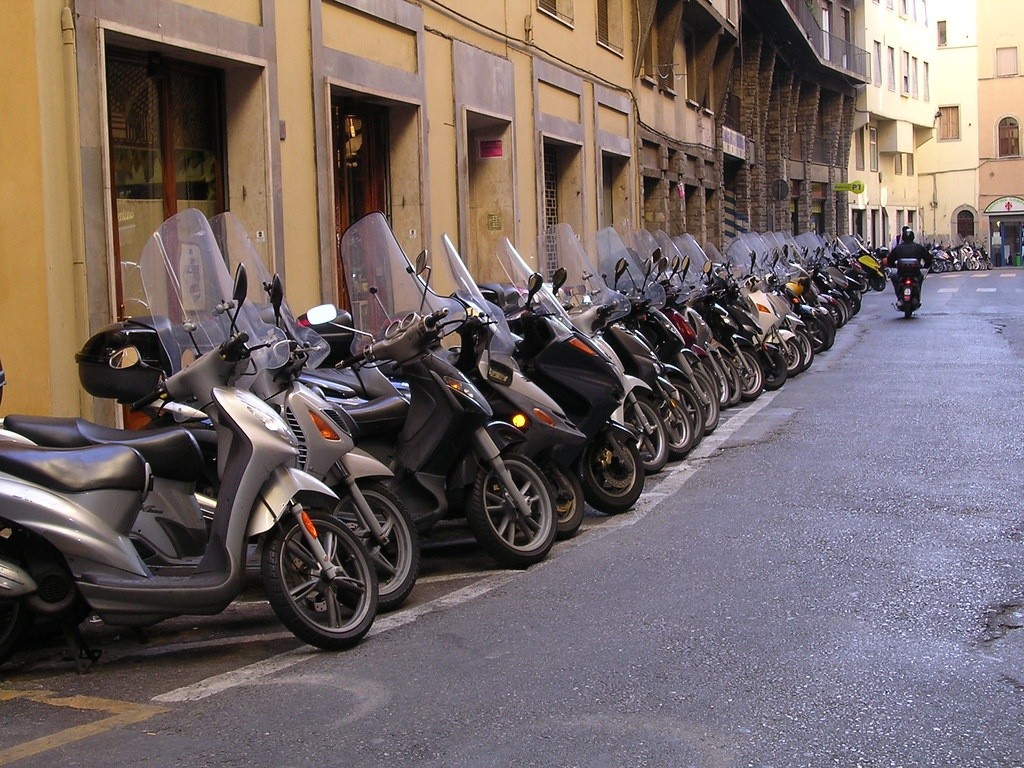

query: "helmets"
(902, 229), (914, 241)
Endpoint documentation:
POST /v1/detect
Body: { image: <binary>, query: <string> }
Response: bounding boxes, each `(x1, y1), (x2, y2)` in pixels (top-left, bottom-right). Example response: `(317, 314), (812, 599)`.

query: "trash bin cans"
(1015, 253), (1021, 267)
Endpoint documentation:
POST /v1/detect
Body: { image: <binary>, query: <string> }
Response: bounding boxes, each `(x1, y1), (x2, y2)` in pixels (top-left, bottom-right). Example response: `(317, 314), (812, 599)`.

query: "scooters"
(0, 209), (995, 615)
(0, 207), (380, 671)
(883, 257), (926, 319)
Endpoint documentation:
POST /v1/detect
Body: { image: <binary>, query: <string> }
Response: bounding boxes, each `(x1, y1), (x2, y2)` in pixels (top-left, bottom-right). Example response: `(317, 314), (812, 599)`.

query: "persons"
(886, 229), (933, 309)
